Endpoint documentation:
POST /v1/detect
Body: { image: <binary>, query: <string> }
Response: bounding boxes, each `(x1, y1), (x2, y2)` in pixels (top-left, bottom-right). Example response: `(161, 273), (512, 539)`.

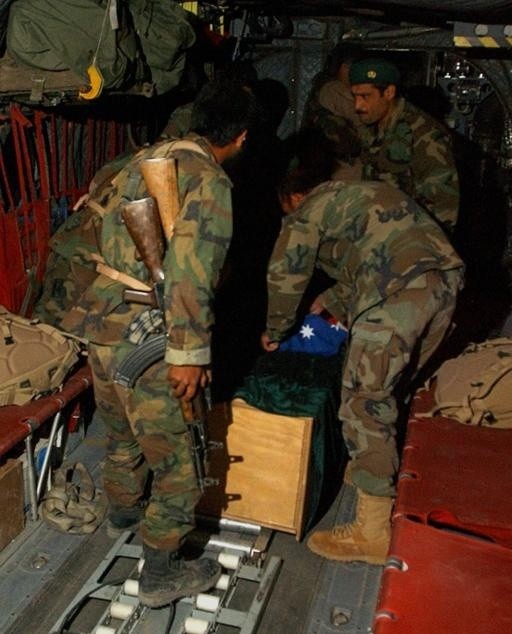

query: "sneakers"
(341, 455), (356, 488)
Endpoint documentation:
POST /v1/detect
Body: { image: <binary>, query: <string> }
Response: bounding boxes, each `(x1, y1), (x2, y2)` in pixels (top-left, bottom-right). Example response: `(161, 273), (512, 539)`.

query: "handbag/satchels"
(1, 1), (141, 108)
(122, 1), (197, 99)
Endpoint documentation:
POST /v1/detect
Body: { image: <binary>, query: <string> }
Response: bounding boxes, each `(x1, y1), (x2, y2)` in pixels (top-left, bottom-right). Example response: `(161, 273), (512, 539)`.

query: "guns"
(114, 157), (224, 496)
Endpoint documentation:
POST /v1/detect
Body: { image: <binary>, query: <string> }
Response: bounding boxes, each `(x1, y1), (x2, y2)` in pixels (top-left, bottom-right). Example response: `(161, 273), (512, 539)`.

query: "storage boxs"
(199, 347), (343, 544)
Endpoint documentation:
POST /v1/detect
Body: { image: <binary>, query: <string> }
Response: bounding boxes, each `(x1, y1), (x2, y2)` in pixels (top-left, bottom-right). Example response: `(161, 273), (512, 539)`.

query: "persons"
(344, 50), (462, 244)
(298, 55), (358, 184)
(260, 158), (468, 568)
(60, 79), (261, 607)
(31, 95), (198, 338)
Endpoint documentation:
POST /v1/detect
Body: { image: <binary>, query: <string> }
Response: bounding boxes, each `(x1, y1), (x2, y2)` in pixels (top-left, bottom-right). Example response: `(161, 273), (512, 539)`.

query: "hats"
(348, 58), (402, 87)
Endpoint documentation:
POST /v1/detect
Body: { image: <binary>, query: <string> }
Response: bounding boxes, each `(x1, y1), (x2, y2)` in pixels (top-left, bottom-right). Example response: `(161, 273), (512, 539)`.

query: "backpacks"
(432, 335), (512, 432)
(0, 304), (89, 408)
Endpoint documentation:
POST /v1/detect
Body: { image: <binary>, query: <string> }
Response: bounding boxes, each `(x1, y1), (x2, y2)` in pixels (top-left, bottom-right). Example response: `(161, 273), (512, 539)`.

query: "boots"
(107, 500), (147, 539)
(138, 543), (223, 609)
(306, 485), (396, 568)
